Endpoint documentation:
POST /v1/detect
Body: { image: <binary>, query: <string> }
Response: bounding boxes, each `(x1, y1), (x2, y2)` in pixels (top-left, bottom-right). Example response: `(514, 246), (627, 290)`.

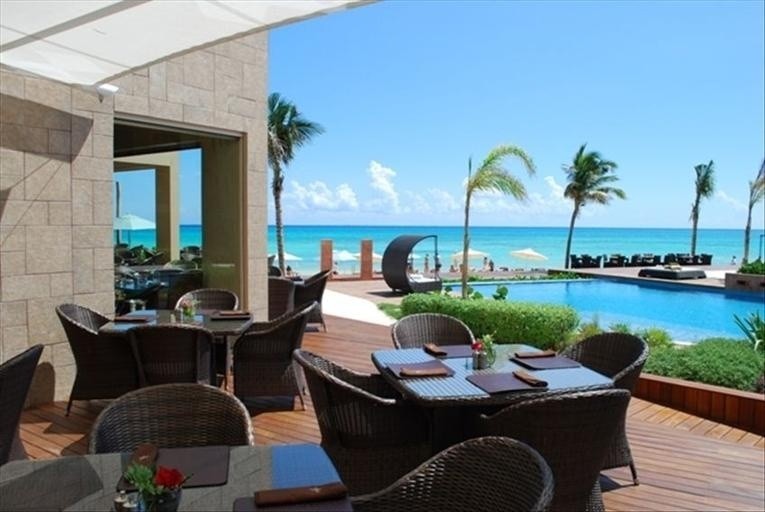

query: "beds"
(509, 247), (548, 261)
(112, 213), (156, 246)
(452, 247), (487, 259)
(315, 248), (384, 264)
(266, 251), (302, 261)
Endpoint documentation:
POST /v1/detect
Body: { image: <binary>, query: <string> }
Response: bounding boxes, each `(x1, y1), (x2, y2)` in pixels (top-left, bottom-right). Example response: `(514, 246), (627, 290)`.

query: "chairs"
(392, 312), (477, 351)
(294, 349), (442, 489)
(293, 271), (331, 332)
(570, 252), (712, 281)
(2, 344), (44, 466)
(350, 436), (554, 511)
(114, 233), (303, 320)
(88, 382), (254, 453)
(57, 304), (138, 416)
(233, 302), (318, 410)
(561, 332), (649, 486)
(471, 389), (632, 510)
(176, 287), (239, 392)
(127, 321), (226, 389)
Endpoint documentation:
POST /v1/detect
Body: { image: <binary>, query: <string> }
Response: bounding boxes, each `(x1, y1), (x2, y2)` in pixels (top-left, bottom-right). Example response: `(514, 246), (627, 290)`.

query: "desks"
(372, 343), (614, 463)
(100, 310), (253, 390)
(2, 441), (352, 511)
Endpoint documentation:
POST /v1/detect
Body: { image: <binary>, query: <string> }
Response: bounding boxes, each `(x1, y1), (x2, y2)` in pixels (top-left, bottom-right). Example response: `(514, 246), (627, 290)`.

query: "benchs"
(382, 235), (442, 295)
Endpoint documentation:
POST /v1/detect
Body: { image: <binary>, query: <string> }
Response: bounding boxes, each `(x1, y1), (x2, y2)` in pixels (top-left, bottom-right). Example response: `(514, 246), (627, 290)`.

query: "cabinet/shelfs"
(471, 348), (488, 371)
(113, 489), (140, 511)
(168, 306), (184, 323)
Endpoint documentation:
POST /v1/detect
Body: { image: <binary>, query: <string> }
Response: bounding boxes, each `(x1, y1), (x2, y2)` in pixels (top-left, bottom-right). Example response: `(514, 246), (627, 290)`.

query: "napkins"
(424, 343), (447, 355)
(123, 442), (160, 482)
(513, 370), (548, 387)
(514, 349), (556, 359)
(255, 482), (348, 508)
(401, 367), (447, 376)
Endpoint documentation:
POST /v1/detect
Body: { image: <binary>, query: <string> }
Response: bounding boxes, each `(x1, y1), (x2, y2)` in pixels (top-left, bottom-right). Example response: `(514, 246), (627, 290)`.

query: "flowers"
(123, 460), (186, 510)
(473, 330), (499, 370)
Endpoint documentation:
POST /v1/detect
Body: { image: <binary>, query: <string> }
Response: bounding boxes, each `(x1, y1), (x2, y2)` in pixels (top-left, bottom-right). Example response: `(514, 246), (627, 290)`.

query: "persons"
(424, 253), (494, 272)
(730, 255), (737, 265)
